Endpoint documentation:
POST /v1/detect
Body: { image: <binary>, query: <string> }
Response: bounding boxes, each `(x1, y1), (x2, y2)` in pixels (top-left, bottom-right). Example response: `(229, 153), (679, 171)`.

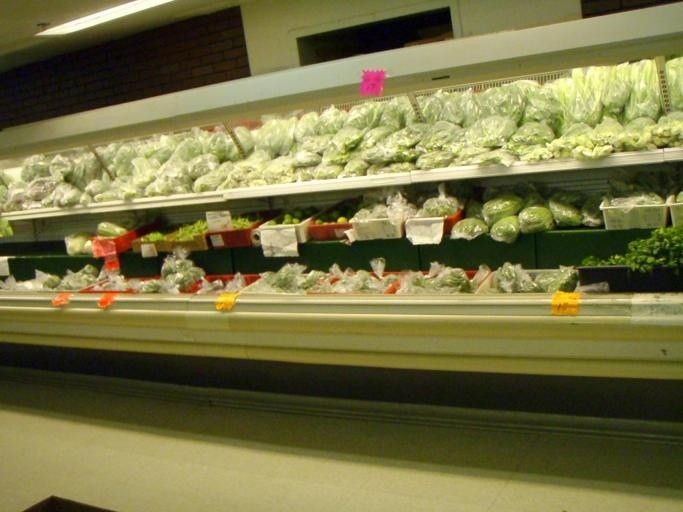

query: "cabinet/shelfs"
(0, 0), (683, 434)
